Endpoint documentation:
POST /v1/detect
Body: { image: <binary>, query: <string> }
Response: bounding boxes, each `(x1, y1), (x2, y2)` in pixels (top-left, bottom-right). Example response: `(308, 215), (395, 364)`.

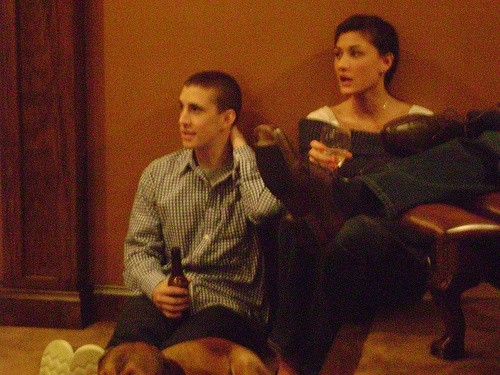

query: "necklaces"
(380, 100), (389, 111)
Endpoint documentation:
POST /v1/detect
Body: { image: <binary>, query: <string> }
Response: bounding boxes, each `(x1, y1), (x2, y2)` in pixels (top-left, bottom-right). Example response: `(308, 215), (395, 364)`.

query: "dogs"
(98, 337), (275, 375)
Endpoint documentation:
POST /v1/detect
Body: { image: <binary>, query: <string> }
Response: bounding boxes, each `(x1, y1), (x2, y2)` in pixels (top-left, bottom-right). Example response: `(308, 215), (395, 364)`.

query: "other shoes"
(39, 340), (74, 375)
(71, 344), (105, 375)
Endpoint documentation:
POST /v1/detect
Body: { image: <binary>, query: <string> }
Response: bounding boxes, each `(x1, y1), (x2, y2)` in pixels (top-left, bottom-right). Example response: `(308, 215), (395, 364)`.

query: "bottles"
(168, 246), (191, 322)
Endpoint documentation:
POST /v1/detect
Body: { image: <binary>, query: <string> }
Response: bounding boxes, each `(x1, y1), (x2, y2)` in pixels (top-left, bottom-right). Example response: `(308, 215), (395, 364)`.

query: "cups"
(321, 126), (353, 173)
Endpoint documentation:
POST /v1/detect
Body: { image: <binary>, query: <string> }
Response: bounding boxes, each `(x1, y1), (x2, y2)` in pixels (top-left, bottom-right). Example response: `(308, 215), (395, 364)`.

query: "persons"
(255, 12), (434, 375)
(39, 70), (285, 375)
